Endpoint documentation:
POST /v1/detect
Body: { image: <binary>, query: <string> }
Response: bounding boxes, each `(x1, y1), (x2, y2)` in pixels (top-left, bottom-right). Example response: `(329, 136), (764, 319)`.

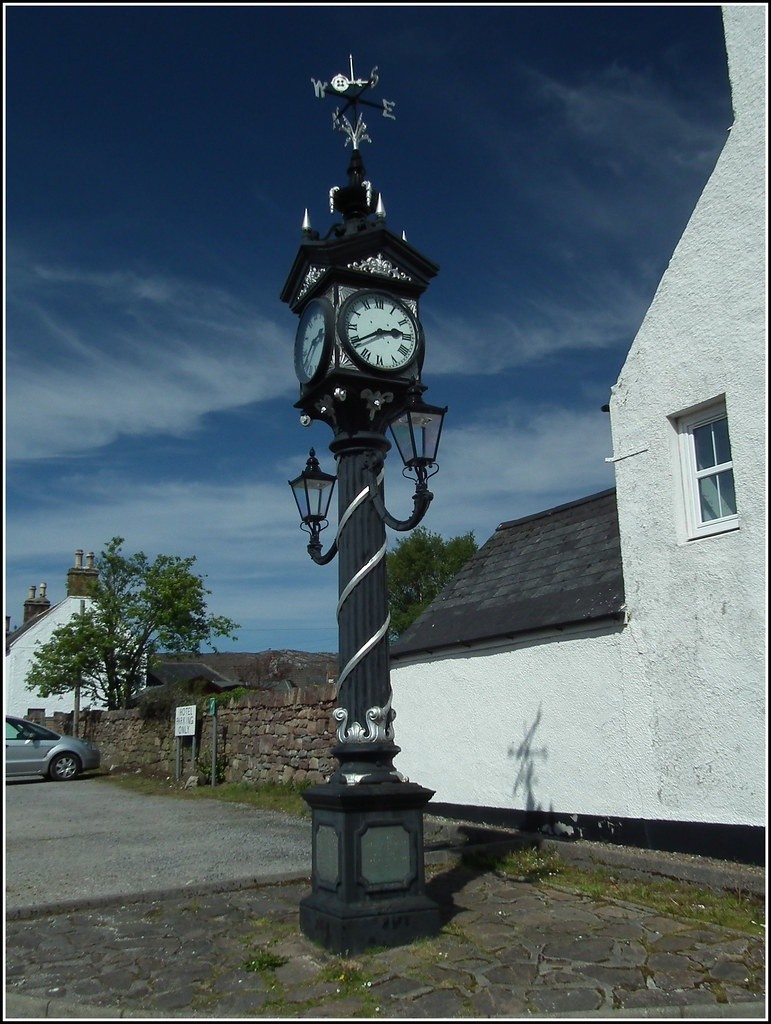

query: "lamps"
(288, 447), (338, 565)
(367, 375), (448, 532)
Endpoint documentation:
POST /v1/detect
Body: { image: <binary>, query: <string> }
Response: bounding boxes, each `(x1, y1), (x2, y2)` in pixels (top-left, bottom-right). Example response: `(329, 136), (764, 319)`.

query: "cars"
(5, 715), (101, 782)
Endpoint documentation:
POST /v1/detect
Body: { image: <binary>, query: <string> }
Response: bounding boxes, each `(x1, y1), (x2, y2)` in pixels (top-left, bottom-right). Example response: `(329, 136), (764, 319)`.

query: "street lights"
(283, 57), (454, 961)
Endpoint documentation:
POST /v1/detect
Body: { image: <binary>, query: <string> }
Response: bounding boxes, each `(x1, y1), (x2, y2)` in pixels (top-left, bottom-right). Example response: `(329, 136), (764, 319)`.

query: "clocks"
(338, 288), (421, 374)
(293, 297), (335, 386)
